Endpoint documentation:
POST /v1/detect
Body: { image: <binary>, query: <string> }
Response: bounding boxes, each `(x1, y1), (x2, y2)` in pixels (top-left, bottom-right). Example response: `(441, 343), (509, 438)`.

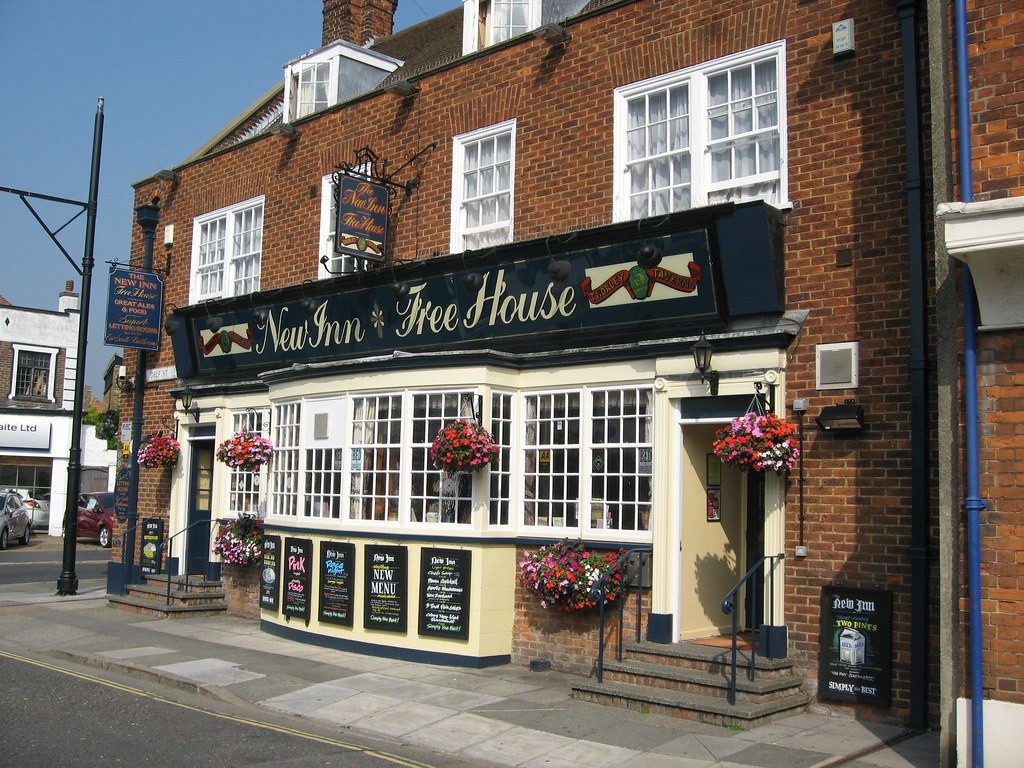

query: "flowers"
(516, 538), (631, 615)
(712, 412), (801, 477)
(136, 435), (181, 469)
(212, 520), (263, 569)
(215, 426), (275, 474)
(430, 417), (501, 480)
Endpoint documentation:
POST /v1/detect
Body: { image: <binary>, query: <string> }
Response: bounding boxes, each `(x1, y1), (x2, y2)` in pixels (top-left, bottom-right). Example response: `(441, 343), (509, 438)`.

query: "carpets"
(689, 632), (760, 650)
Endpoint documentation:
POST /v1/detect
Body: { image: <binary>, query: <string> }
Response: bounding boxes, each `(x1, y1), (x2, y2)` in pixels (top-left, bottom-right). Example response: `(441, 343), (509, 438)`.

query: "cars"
(8, 488), (50, 530)
(0, 491), (31, 549)
(61, 490), (114, 547)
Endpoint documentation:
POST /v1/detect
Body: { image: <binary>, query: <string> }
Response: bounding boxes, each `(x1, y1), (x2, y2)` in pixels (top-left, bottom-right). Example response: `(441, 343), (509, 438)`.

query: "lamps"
(320, 256), (357, 274)
(164, 302), (196, 336)
(461, 246), (496, 295)
(268, 123), (302, 140)
(689, 329), (720, 396)
(249, 288), (284, 328)
(531, 23), (572, 47)
(545, 232), (578, 283)
(180, 384), (201, 423)
(383, 79), (422, 98)
(153, 169), (182, 187)
(813, 398), (868, 433)
(204, 297), (236, 333)
(390, 260), (427, 300)
(636, 215), (671, 271)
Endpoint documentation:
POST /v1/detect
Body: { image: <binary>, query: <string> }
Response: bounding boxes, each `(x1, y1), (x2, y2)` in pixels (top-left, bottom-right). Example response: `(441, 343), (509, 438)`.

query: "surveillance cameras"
(118, 366), (126, 380)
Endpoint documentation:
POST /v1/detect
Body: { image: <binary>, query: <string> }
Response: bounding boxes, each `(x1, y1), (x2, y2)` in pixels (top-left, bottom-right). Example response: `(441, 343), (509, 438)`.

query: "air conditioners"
(299, 278), (336, 316)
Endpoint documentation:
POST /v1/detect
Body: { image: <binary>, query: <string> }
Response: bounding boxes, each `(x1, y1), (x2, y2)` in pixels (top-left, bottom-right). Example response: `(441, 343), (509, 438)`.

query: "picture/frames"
(706, 488), (722, 522)
(706, 452), (722, 486)
